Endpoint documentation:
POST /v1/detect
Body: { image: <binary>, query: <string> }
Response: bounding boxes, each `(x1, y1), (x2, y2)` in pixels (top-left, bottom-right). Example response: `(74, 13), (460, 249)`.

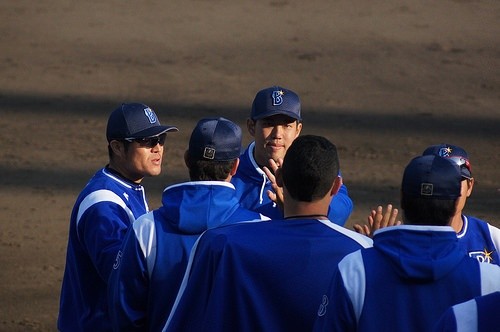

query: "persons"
(316, 155), (500, 332)
(106, 117), (271, 332)
(353, 143), (499, 264)
(160, 135), (374, 332)
(57, 103), (180, 332)
(229, 85), (353, 228)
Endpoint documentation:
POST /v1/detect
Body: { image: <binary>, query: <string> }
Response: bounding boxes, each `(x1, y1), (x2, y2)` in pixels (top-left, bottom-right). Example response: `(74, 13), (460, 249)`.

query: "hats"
(106, 103), (179, 137)
(189, 118), (243, 161)
(251, 87), (301, 121)
(422, 143), (471, 180)
(402, 154), (462, 199)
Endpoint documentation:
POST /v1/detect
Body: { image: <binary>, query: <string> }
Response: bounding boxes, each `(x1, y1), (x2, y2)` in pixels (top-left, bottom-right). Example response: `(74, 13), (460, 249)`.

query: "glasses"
(118, 132), (167, 147)
(446, 156), (472, 173)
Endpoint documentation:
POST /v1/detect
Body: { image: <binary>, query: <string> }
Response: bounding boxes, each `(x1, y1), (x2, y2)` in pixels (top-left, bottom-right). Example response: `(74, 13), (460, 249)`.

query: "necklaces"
(283, 214), (330, 220)
